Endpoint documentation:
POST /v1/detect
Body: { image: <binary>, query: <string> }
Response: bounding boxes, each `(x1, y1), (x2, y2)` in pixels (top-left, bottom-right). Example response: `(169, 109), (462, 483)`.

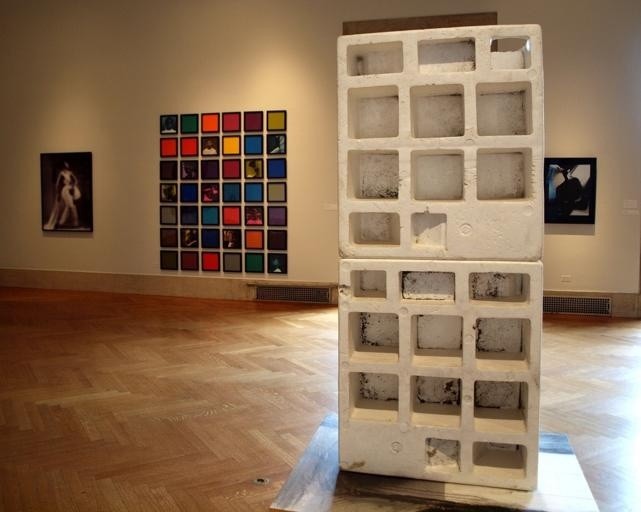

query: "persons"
(52, 158), (82, 229)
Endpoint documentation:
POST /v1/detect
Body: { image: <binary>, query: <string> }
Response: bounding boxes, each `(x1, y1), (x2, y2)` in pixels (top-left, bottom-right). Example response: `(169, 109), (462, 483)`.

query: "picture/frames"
(39, 151), (95, 234)
(544, 156), (599, 226)
(159, 109), (289, 276)
